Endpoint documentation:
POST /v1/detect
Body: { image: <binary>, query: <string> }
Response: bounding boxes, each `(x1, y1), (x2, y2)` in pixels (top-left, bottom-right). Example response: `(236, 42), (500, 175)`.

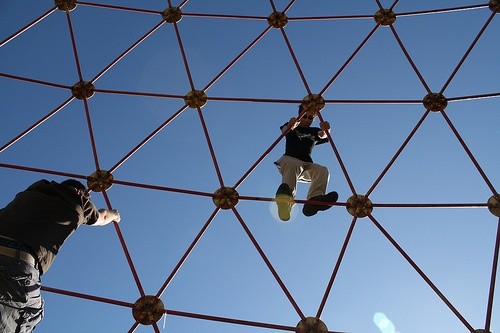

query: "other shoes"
(302, 191), (338, 217)
(276, 183), (291, 221)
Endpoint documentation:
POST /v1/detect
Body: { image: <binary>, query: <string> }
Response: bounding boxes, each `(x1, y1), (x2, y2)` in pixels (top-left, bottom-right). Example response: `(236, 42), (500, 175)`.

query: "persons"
(274, 103), (338, 222)
(0, 179), (121, 333)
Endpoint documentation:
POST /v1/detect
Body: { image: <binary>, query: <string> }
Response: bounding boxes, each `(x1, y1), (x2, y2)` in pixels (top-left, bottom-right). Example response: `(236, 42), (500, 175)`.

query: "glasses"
(301, 114), (314, 120)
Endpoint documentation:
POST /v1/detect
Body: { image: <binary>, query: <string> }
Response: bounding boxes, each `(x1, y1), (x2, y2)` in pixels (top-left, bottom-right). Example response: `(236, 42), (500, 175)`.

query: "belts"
(0, 246), (38, 267)
